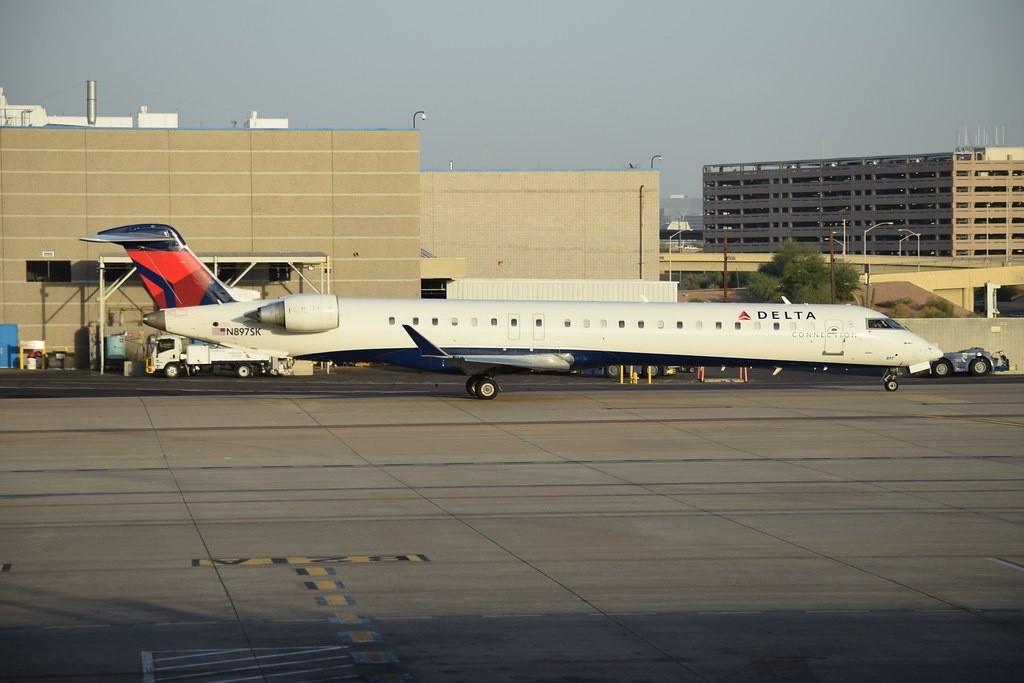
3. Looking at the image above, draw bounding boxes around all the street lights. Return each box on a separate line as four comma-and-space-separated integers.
413, 111, 426, 129
898, 229, 920, 258
670, 230, 682, 281
899, 234, 921, 256
986, 204, 990, 255
843, 220, 847, 254
864, 222, 894, 264
651, 155, 662, 169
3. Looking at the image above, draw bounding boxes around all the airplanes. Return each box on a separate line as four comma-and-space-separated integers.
79, 223, 944, 400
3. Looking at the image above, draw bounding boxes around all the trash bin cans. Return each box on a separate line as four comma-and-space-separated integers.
34, 350, 42, 368
27, 351, 36, 370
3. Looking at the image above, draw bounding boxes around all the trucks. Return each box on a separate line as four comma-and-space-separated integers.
154, 335, 269, 378
